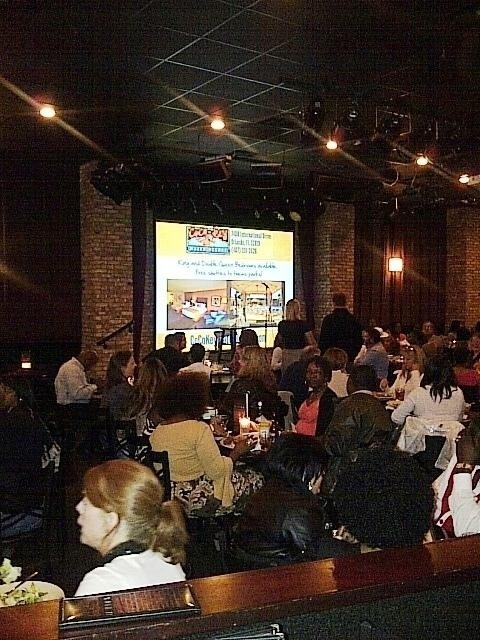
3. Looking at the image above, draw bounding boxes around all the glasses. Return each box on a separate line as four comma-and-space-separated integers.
403, 347, 415, 351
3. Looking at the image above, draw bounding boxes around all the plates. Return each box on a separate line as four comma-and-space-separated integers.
220, 438, 239, 450
0, 581, 64, 606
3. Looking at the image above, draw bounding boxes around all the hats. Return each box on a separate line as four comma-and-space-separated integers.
374, 326, 389, 338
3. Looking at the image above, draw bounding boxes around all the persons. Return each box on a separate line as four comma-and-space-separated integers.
380, 345, 427, 403
317, 293, 364, 362
274, 298, 318, 372
174, 332, 186, 352
323, 449, 436, 549
430, 403, 480, 539
295, 356, 338, 437
324, 365, 398, 456
463, 334, 480, 369
125, 358, 165, 434
323, 348, 352, 398
177, 343, 211, 395
100, 351, 137, 421
0, 372, 46, 539
73, 458, 188, 598
229, 330, 259, 374
52, 350, 98, 430
142, 334, 191, 374
227, 433, 326, 572
421, 320, 446, 358
220, 346, 283, 434
389, 356, 463, 455
148, 373, 265, 518
354, 329, 389, 381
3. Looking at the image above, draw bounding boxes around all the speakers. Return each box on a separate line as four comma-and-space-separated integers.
88, 170, 128, 206
248, 162, 284, 189
305, 172, 359, 205
196, 157, 233, 183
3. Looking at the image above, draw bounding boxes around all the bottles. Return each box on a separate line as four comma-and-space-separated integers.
255, 400, 264, 424
271, 411, 279, 443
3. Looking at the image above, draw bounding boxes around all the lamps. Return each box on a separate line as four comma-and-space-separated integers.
250, 162, 288, 188
371, 106, 407, 146
272, 190, 323, 223
330, 107, 359, 144
307, 171, 349, 200
90, 171, 145, 205
196, 157, 234, 183
366, 198, 398, 225
303, 98, 328, 141
381, 168, 400, 186
425, 118, 460, 151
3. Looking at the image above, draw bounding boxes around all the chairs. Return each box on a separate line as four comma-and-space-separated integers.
106, 418, 147, 457
119, 438, 128, 439
137, 451, 169, 498
1, 461, 55, 577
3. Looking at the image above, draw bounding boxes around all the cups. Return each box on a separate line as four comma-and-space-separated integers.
396, 385, 405, 401
260, 423, 270, 442
239, 417, 250, 433
